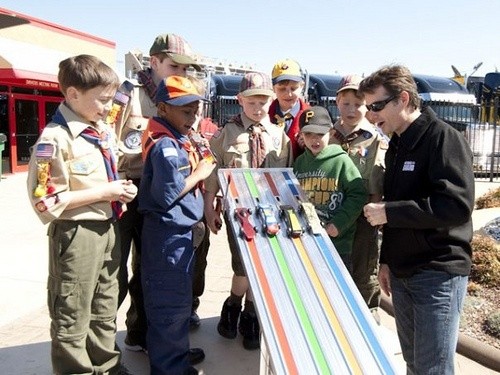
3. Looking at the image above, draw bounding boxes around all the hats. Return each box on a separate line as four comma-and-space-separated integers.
150, 31, 201, 72
240, 72, 273, 98
298, 106, 333, 134
335, 75, 362, 94
271, 59, 303, 85
154, 75, 212, 107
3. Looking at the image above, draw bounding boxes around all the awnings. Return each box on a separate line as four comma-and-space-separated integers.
0, 37, 79, 92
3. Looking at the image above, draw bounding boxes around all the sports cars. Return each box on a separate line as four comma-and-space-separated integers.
233, 206, 259, 242
255, 205, 281, 237
278, 204, 305, 239
298, 201, 324, 236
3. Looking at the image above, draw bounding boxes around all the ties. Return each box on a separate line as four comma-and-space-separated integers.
235, 114, 265, 169
51, 109, 123, 223
333, 128, 363, 154
273, 113, 292, 129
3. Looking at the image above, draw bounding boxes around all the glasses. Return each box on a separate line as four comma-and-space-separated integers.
366, 93, 396, 112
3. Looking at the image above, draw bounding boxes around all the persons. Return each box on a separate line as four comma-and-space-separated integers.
207, 72, 294, 349
136, 75, 217, 375
358, 65, 475, 374
103, 33, 201, 355
290, 106, 366, 278
267, 56, 312, 162
191, 118, 220, 326
27, 54, 139, 375
328, 74, 389, 325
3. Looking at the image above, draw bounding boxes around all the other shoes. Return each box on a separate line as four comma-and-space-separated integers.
191, 311, 200, 326
238, 313, 260, 351
189, 347, 206, 363
125, 335, 148, 354
217, 296, 242, 339
109, 365, 134, 375
185, 365, 199, 375
